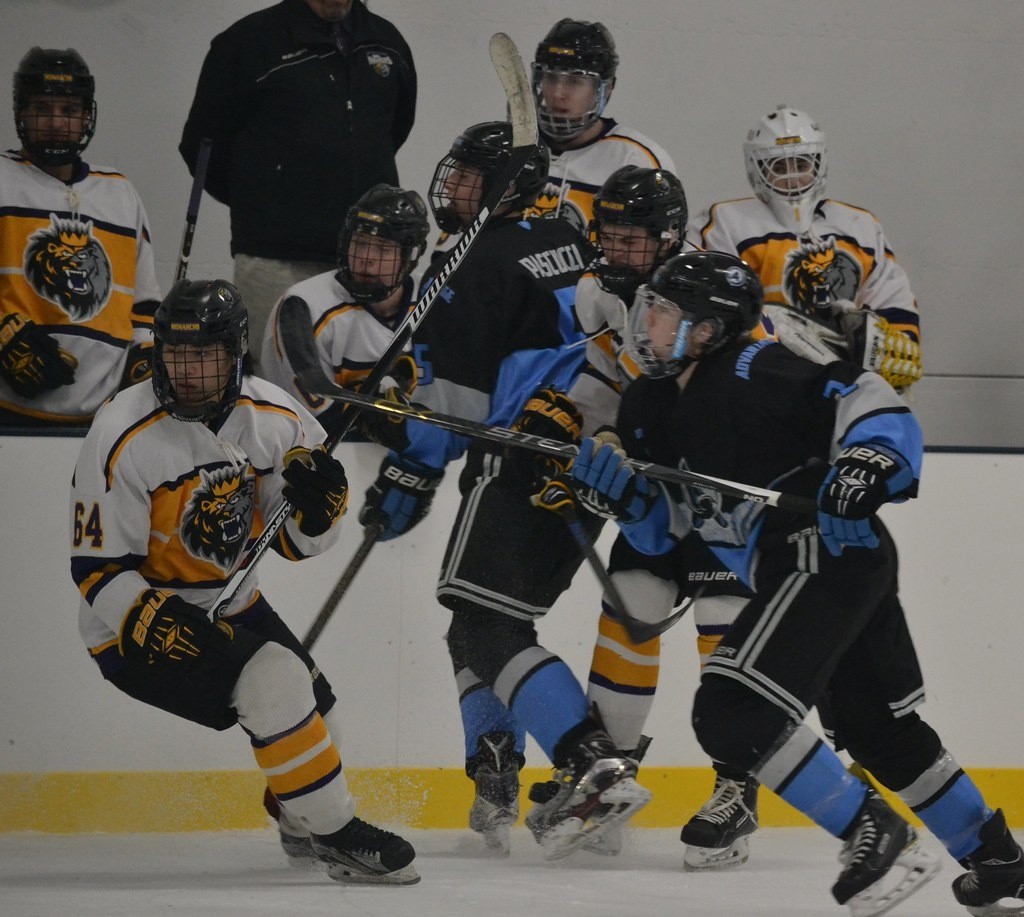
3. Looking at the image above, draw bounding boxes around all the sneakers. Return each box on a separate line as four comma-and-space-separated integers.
950, 807, 1024, 917
469, 731, 525, 858
536, 708, 652, 867
832, 762, 943, 917
680, 760, 761, 873
527, 734, 653, 856
263, 786, 421, 886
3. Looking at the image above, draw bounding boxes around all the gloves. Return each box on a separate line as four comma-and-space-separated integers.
359, 449, 446, 542
500, 385, 586, 499
569, 435, 659, 525
818, 445, 914, 557
1, 311, 77, 400
124, 298, 162, 384
119, 587, 237, 690
281, 445, 349, 537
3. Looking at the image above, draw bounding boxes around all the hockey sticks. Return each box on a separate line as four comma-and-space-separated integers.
277, 292, 819, 514
204, 34, 539, 624
302, 523, 382, 653
175, 138, 213, 281
559, 505, 706, 643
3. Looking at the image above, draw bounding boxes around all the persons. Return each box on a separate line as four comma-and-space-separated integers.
0, 45, 162, 435
362, 110, 654, 856
514, 9, 683, 253
175, 0, 421, 360
256, 179, 431, 439
511, 158, 1024, 917
680, 104, 921, 397
67, 281, 421, 886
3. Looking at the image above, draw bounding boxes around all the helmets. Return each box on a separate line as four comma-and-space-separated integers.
593, 166, 687, 294
153, 279, 249, 422
526, 18, 619, 140
745, 105, 826, 234
11, 46, 98, 164
338, 185, 429, 303
428, 121, 549, 233
622, 250, 764, 379
